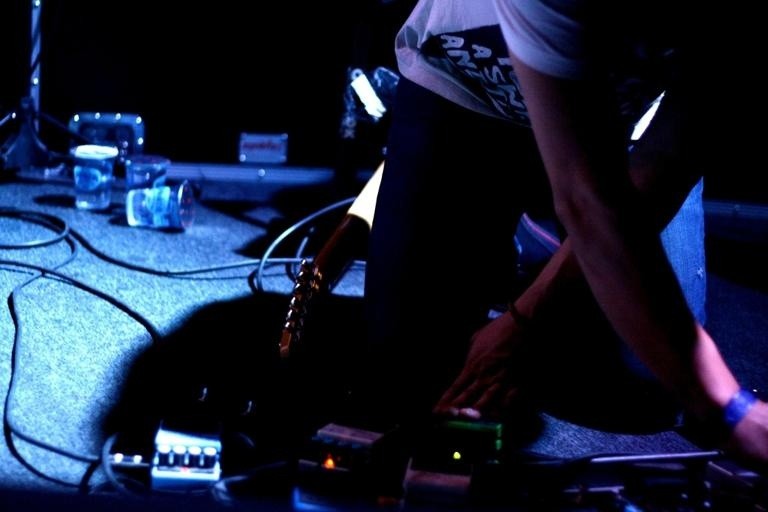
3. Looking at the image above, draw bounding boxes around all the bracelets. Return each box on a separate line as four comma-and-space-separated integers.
501, 305, 543, 340
711, 387, 759, 445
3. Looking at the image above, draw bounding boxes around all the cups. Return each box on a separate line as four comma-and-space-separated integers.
125, 154, 172, 192
125, 180, 199, 232
70, 142, 119, 211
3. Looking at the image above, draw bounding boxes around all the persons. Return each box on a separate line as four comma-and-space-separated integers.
364, 1, 767, 473
